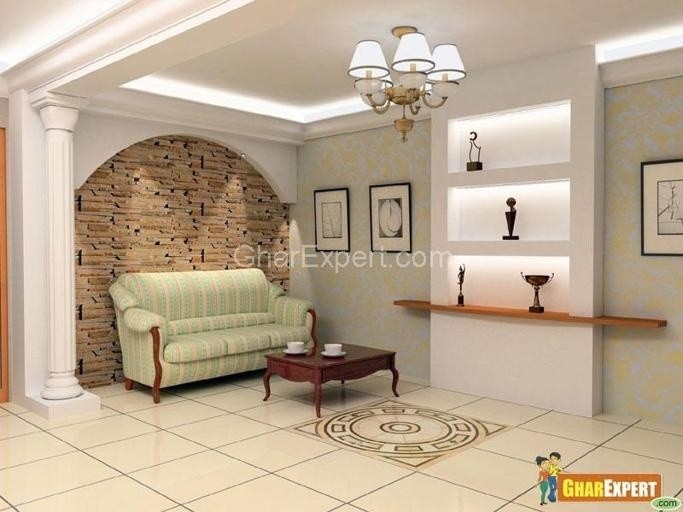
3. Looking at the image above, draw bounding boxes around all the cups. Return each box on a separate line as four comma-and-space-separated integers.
286, 342, 304, 353
323, 344, 343, 355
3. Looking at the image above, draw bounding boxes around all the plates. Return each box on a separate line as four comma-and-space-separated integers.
281, 349, 308, 355
319, 352, 346, 358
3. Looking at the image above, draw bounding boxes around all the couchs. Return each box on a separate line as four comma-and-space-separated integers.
107, 266, 316, 404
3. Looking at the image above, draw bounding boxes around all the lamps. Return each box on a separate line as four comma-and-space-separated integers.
350, 24, 468, 139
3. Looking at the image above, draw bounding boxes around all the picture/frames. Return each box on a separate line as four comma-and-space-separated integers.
312, 187, 352, 253
639, 159, 683, 258
368, 182, 413, 253
